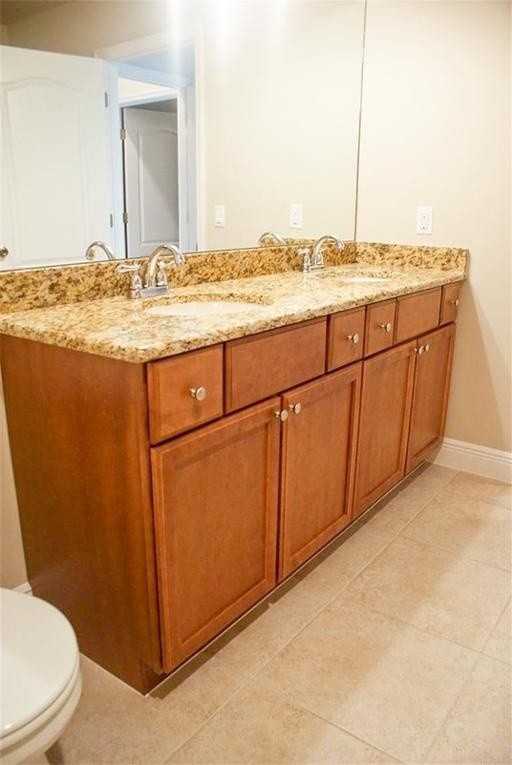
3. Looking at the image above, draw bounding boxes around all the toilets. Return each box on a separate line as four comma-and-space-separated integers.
0, 584, 83, 765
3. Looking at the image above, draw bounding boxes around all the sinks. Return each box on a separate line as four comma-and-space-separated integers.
142, 294, 271, 318
319, 269, 399, 286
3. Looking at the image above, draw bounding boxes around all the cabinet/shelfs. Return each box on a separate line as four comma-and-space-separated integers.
353, 279, 465, 524
1, 306, 366, 701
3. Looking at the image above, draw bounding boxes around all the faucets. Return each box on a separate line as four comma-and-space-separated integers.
313, 235, 346, 264
259, 232, 284, 245
144, 245, 184, 287
85, 241, 115, 262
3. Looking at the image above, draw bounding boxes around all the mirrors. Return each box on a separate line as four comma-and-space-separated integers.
0, 0, 369, 312
